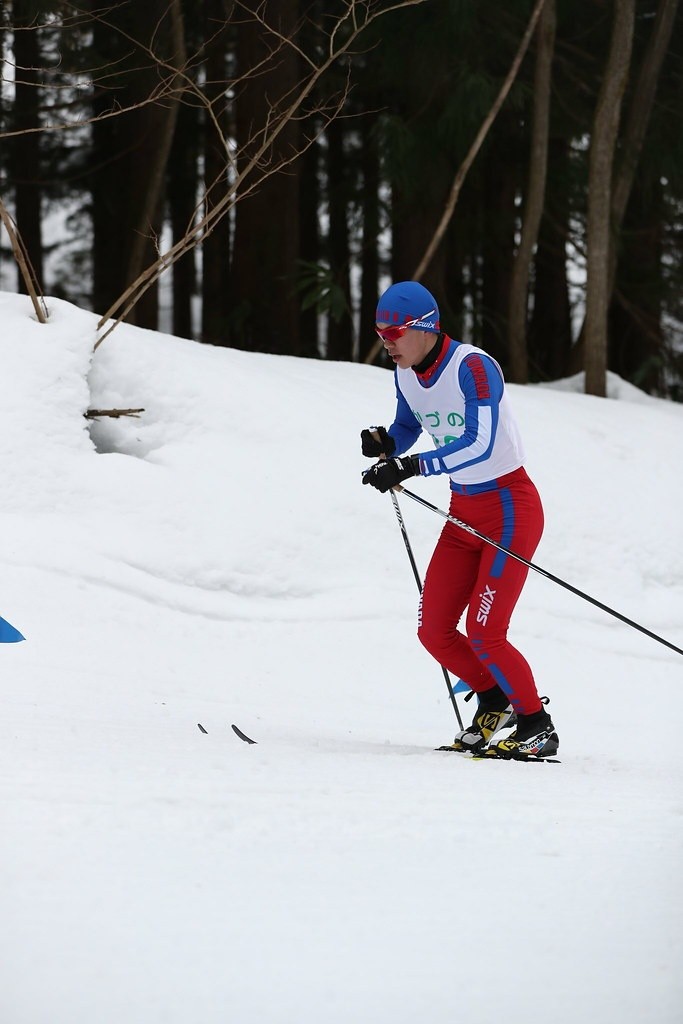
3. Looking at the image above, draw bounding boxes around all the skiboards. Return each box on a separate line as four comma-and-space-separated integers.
199, 720, 562, 766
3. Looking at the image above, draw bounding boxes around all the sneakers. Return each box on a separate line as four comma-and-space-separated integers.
454, 702, 518, 751
488, 714, 559, 757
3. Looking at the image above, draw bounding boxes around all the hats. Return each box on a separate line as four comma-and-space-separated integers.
375, 281, 440, 334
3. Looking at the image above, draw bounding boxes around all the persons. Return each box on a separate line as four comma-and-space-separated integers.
360, 281, 560, 760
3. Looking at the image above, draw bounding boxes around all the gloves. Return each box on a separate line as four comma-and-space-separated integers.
361, 426, 396, 458
362, 456, 417, 493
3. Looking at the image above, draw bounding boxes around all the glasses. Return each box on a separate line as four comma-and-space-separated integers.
374, 323, 410, 343
